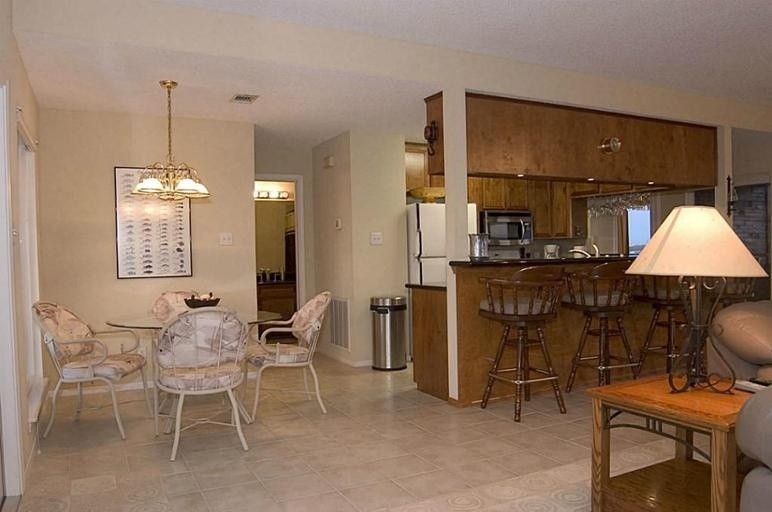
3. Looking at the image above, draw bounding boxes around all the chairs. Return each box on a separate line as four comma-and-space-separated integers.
705, 254, 765, 326
251, 291, 332, 423
153, 291, 200, 316
476, 265, 570, 422
156, 307, 249, 461
562, 273, 641, 393
32, 301, 154, 440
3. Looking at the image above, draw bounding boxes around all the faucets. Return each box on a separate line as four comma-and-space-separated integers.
569, 244, 599, 258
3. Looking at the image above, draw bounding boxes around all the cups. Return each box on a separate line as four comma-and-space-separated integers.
468, 233, 489, 256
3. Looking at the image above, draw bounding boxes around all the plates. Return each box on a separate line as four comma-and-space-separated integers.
469, 256, 490, 263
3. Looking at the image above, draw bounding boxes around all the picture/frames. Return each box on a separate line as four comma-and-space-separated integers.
114, 166, 192, 279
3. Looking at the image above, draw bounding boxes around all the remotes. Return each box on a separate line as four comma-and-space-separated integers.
735, 379, 767, 393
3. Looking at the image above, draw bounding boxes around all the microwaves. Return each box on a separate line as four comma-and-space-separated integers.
481, 210, 534, 245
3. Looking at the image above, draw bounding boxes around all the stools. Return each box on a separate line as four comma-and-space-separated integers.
635, 275, 683, 377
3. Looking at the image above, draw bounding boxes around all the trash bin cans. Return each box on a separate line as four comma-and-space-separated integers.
370, 295, 407, 371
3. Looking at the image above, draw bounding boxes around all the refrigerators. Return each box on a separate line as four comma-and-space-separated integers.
408, 201, 478, 286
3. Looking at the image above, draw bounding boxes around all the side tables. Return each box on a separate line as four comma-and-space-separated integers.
584, 371, 759, 512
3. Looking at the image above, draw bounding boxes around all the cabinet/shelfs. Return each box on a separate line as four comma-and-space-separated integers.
452, 250, 692, 406
571, 180, 599, 197
257, 281, 297, 339
601, 183, 671, 196
469, 174, 528, 210
405, 284, 448, 401
528, 177, 572, 240
402, 140, 445, 195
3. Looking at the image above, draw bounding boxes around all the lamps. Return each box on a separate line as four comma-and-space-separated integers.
625, 206, 770, 393
130, 79, 211, 200
587, 187, 650, 219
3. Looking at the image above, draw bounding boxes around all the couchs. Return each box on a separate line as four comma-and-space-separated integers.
736, 386, 772, 512
706, 298, 772, 385
